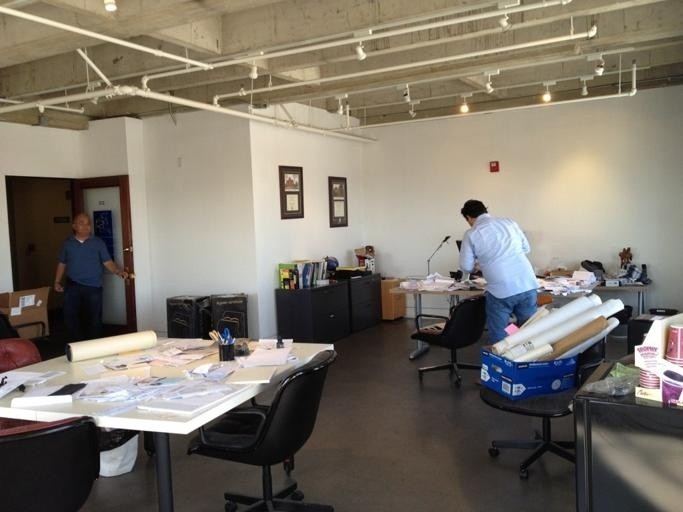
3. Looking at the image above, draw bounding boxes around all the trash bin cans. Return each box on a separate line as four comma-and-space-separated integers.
605, 326, 628, 363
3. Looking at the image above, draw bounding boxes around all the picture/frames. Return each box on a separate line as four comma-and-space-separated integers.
326, 176, 348, 227
278, 165, 304, 221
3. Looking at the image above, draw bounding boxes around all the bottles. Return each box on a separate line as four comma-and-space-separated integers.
642, 264, 648, 279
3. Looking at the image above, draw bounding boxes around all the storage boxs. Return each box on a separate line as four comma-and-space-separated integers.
478, 345, 577, 400
0, 284, 52, 341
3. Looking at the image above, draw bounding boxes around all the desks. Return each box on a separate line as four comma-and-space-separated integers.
0, 336, 337, 512
536, 284, 650, 342
389, 283, 489, 360
571, 349, 682, 511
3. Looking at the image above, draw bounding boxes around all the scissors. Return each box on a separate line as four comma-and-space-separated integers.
209, 328, 231, 345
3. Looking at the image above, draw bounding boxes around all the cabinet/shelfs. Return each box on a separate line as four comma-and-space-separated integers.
274, 281, 349, 344
379, 275, 406, 321
332, 270, 382, 333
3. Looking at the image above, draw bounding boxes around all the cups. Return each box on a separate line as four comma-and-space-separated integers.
661, 369, 683, 408
218, 343, 235, 362
639, 366, 659, 389
665, 325, 683, 367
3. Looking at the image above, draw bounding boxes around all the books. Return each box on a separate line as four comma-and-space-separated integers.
227, 366, 277, 384
279, 261, 328, 290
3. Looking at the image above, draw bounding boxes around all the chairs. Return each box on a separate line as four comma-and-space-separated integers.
0, 335, 42, 373
478, 355, 605, 481
185, 350, 338, 511
409, 294, 488, 388
0, 415, 101, 512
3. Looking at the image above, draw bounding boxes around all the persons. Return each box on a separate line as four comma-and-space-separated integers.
459, 200, 541, 345
54, 214, 128, 354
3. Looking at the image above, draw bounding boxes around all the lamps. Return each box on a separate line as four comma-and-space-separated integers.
425, 235, 450, 276
246, 8, 516, 85
330, 75, 597, 123
